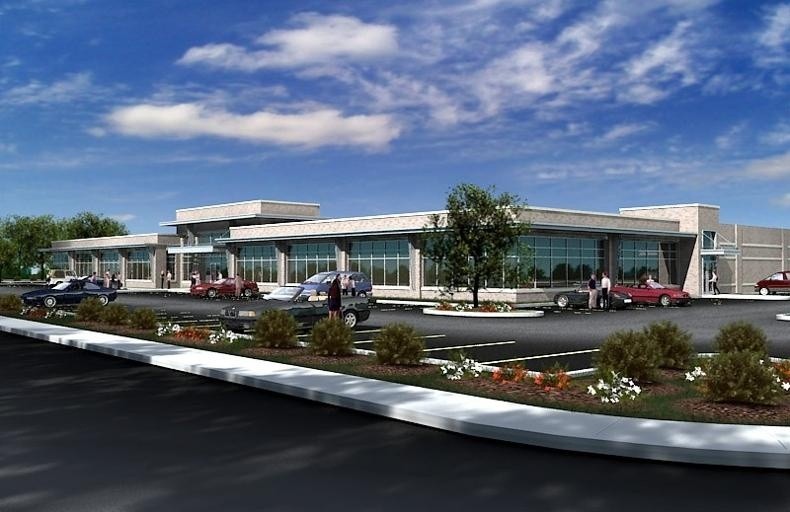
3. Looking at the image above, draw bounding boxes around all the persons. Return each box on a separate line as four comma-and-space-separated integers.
160, 270, 164, 289
646, 273, 654, 283
638, 277, 648, 289
190, 270, 195, 287
217, 271, 222, 280
88, 268, 122, 290
708, 270, 721, 295
46, 270, 52, 285
601, 272, 611, 312
327, 272, 356, 322
192, 270, 201, 285
588, 273, 599, 312
166, 269, 171, 289
234, 273, 243, 299
